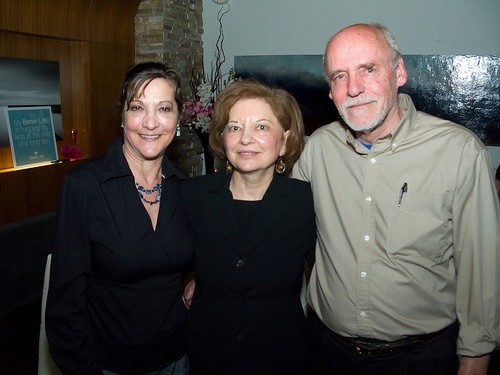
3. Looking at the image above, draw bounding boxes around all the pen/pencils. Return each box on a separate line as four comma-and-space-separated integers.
398, 182, 408, 208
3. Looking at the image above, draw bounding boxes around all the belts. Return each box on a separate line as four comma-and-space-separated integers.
334, 321, 460, 361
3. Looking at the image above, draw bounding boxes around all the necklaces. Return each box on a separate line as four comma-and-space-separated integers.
134, 181, 163, 206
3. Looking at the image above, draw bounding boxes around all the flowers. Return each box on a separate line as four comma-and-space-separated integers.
178, 2, 245, 135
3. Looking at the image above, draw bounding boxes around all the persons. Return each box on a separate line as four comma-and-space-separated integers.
183, 23, 500, 375
44, 62, 193, 375
175, 82, 318, 374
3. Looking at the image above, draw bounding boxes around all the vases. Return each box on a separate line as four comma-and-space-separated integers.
190, 124, 217, 177
